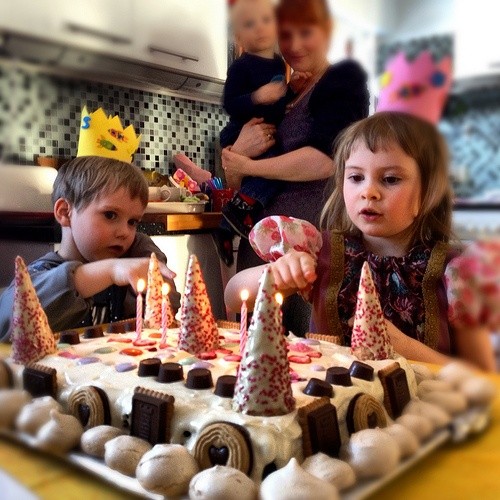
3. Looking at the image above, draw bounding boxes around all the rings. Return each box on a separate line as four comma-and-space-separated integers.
225, 166, 227, 171
268, 134, 273, 141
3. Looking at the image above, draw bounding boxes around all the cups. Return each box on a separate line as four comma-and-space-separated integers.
148, 187, 192, 202
214, 189, 232, 212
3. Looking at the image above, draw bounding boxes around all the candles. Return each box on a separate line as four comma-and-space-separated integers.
240, 289, 250, 348
274, 292, 283, 332
135, 278, 145, 337
161, 282, 171, 343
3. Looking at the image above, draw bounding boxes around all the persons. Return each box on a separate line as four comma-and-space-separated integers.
223, 111, 499, 379
219, 0, 370, 339
0, 156, 181, 344
210, 1, 312, 269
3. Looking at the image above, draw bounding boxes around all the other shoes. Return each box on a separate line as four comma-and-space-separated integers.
220, 193, 254, 239
209, 226, 234, 268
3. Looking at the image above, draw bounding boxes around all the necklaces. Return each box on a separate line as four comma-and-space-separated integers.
287, 68, 324, 109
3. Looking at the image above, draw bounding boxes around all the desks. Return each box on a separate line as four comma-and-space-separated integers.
0, 317, 500, 500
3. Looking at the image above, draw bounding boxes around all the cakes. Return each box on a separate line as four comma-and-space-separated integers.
0, 251, 492, 500
